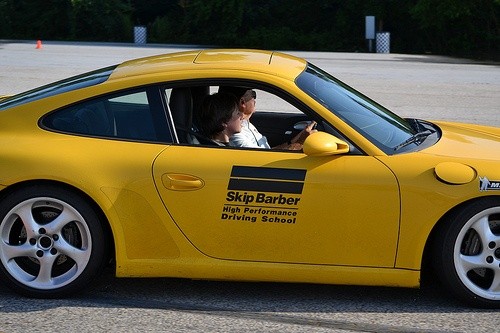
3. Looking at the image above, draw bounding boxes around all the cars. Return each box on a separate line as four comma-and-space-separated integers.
0, 48, 500, 310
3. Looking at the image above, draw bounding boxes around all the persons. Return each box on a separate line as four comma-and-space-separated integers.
218, 87, 317, 149
199, 92, 244, 147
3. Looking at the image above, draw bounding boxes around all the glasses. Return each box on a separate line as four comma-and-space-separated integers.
252, 90, 257, 99
